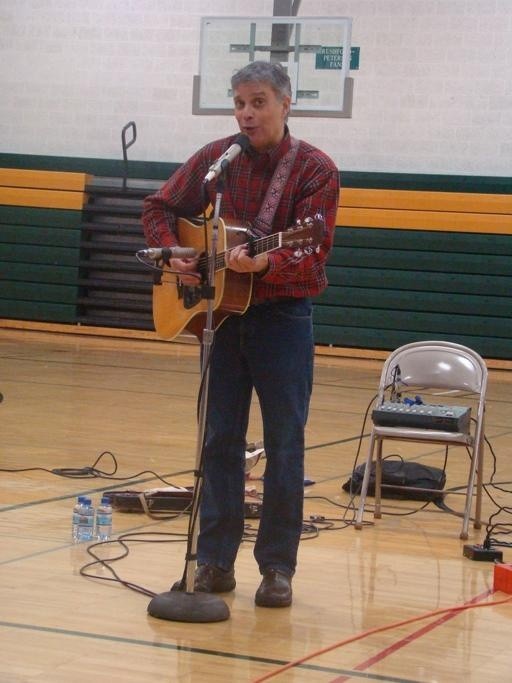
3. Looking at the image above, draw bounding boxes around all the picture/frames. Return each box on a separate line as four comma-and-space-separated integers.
192, 13, 355, 120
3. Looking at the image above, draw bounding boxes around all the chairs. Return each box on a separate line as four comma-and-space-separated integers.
355, 340, 489, 541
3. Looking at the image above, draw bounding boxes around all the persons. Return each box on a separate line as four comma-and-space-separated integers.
139, 59, 341, 608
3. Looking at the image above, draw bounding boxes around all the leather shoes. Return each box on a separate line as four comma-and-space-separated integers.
255, 569, 294, 608
172, 562, 236, 595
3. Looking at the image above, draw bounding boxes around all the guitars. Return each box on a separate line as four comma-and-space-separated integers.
152, 213, 326, 342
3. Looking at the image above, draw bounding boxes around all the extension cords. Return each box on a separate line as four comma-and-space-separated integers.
462, 544, 503, 563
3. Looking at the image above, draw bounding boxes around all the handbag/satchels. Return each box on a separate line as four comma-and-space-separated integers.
343, 459, 448, 501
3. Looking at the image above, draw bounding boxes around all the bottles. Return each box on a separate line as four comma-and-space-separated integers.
73, 498, 86, 538
77, 499, 94, 540
96, 497, 113, 541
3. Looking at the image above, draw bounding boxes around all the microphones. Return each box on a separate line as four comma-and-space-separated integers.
148, 248, 196, 260
201, 133, 252, 186
